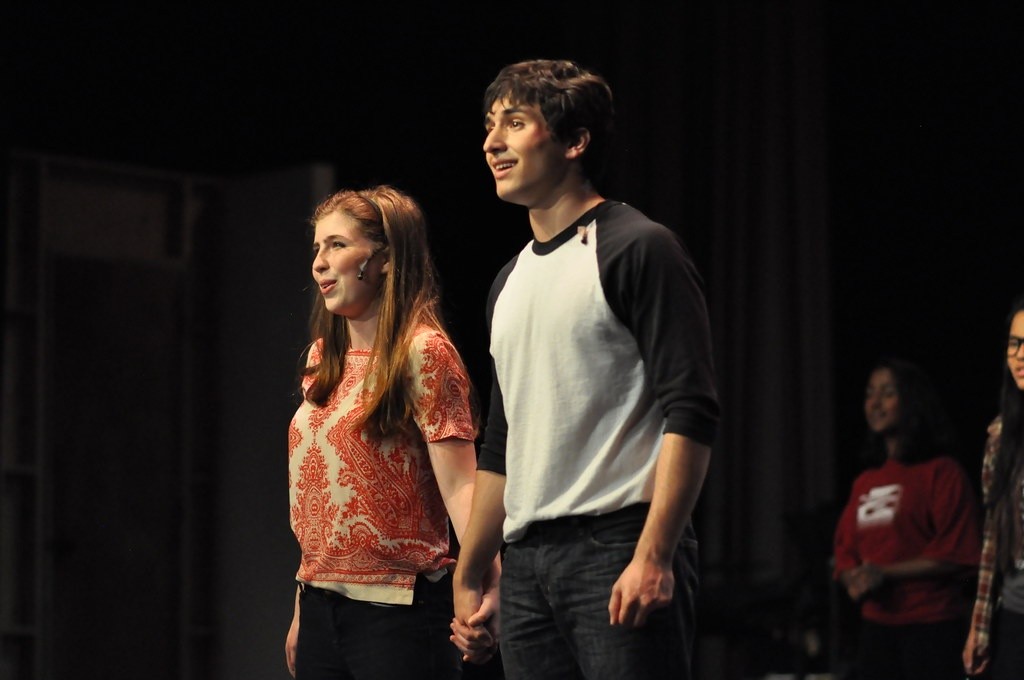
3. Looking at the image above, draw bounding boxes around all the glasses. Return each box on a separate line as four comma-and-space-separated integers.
1006, 336, 1024, 356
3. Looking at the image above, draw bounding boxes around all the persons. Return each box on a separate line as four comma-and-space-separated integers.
830, 311, 1024, 680
282, 60, 718, 680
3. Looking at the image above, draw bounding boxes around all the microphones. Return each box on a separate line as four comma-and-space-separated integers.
358, 247, 384, 280
582, 235, 587, 245
